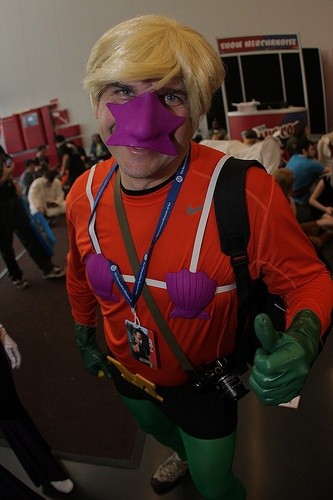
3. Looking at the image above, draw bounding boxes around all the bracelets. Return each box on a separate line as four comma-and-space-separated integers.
0, 324, 6, 337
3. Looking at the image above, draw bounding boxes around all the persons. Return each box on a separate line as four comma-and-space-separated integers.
0, 120, 331, 292
0, 323, 85, 500
65, 14, 333, 500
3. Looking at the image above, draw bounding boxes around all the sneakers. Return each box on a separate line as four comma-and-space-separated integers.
13, 278, 29, 289
151, 452, 190, 490
42, 264, 65, 278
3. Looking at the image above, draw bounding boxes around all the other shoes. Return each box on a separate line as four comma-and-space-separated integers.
51, 478, 74, 492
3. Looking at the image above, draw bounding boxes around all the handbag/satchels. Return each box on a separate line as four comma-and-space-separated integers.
214, 157, 333, 367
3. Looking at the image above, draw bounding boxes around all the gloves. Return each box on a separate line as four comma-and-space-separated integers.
0, 325, 21, 368
73, 321, 113, 379
248, 309, 322, 407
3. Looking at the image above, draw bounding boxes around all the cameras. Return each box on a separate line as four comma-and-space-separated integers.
191, 354, 245, 400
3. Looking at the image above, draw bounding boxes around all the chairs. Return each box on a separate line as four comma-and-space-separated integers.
289, 175, 318, 223
